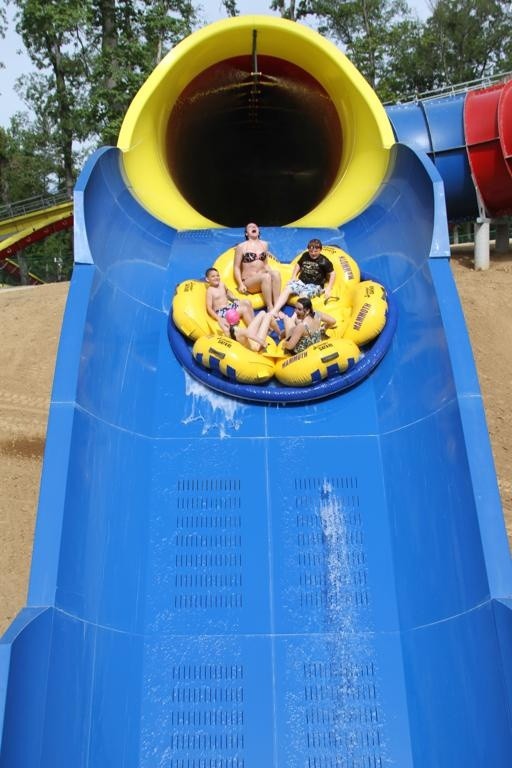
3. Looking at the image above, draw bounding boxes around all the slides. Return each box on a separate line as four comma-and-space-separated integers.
0, 144, 511, 768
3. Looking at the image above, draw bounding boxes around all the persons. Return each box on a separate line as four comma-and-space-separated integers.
225, 308, 284, 354
278, 297, 336, 354
233, 221, 287, 321
268, 238, 335, 319
204, 267, 254, 334
